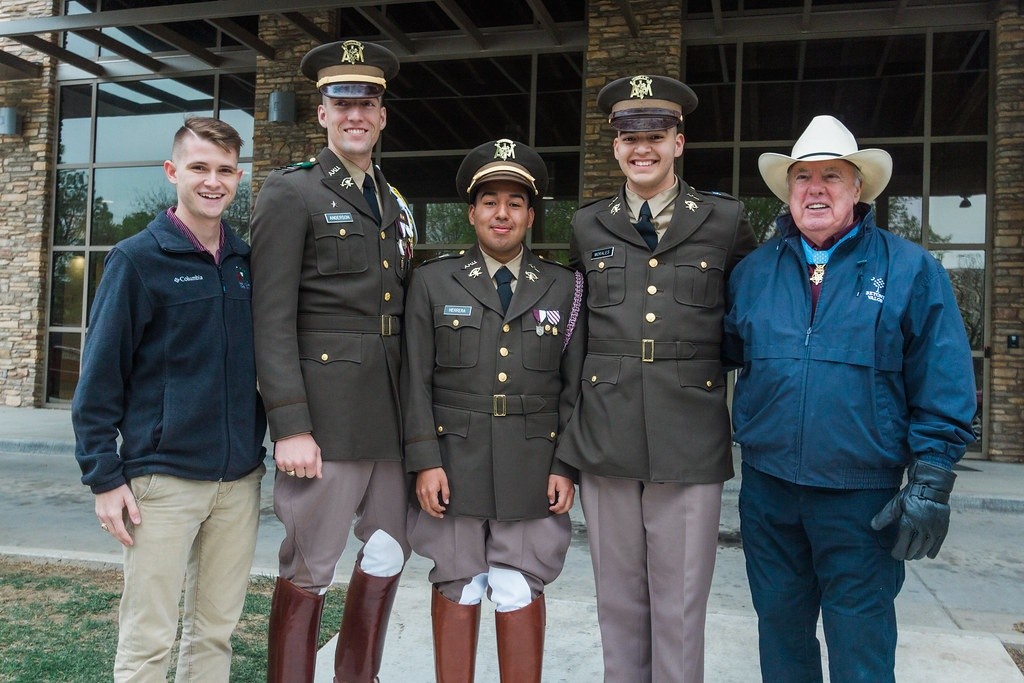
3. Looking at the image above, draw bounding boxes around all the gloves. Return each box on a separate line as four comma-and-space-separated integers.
870, 460, 957, 560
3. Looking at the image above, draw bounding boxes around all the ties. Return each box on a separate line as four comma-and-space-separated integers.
494, 266, 513, 315
362, 173, 382, 227
632, 201, 658, 252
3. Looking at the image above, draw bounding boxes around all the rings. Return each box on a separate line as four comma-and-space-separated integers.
101, 523, 107, 531
287, 470, 294, 475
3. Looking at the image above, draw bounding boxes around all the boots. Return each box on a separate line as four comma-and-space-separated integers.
431, 584, 481, 683
334, 563, 403, 683
496, 595, 547, 683
268, 577, 324, 683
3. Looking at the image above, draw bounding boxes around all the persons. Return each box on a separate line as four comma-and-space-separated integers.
248, 40, 412, 683
568, 75, 760, 683
400, 138, 588, 683
72, 117, 268, 683
725, 115, 978, 683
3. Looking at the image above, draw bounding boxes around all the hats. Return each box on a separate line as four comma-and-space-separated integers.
596, 75, 698, 132
300, 40, 400, 98
759, 115, 893, 205
456, 139, 549, 204
